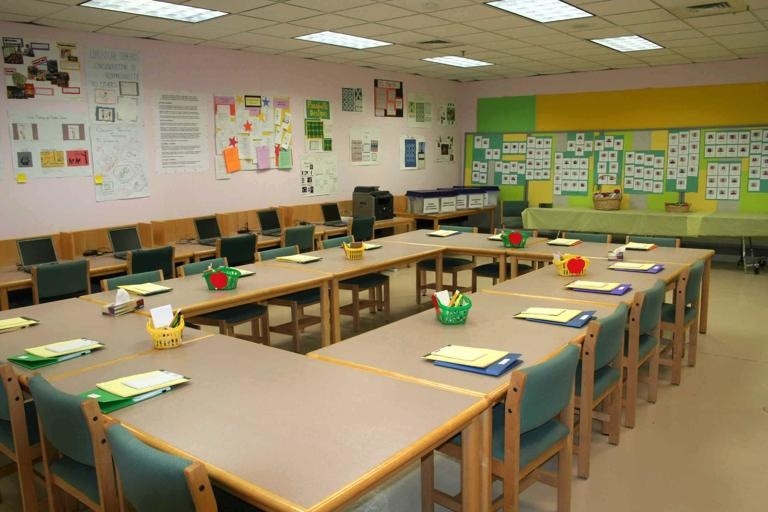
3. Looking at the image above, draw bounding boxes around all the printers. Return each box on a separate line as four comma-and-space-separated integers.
352, 186, 394, 220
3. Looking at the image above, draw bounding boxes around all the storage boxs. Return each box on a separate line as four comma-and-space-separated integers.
452, 185, 500, 208
406, 190, 458, 213
436, 187, 486, 208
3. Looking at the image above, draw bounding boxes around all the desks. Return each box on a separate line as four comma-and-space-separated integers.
0, 296, 216, 387
47, 335, 488, 510
398, 207, 494, 234
80, 243, 450, 351
521, 208, 768, 276
307, 247, 724, 437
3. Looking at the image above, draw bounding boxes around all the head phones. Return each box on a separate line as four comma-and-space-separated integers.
299, 221, 307, 225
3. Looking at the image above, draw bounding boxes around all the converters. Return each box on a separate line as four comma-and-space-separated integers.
83, 248, 97, 257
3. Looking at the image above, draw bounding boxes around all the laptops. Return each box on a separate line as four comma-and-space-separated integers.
107, 225, 144, 260
255, 207, 283, 236
16, 235, 62, 274
192, 215, 222, 246
320, 202, 347, 227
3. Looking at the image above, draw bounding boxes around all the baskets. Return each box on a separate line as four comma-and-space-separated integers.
204, 266, 241, 290
432, 292, 472, 325
593, 198, 621, 210
552, 254, 590, 277
665, 203, 689, 212
500, 230, 528, 248
147, 309, 184, 350
343, 242, 364, 260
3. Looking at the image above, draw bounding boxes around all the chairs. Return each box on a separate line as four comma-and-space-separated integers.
572, 300, 635, 481
13, 215, 376, 311
623, 278, 669, 429
28, 371, 120, 511
411, 223, 682, 312
416, 336, 585, 511
103, 418, 219, 511
659, 260, 706, 387
1, 359, 43, 510
